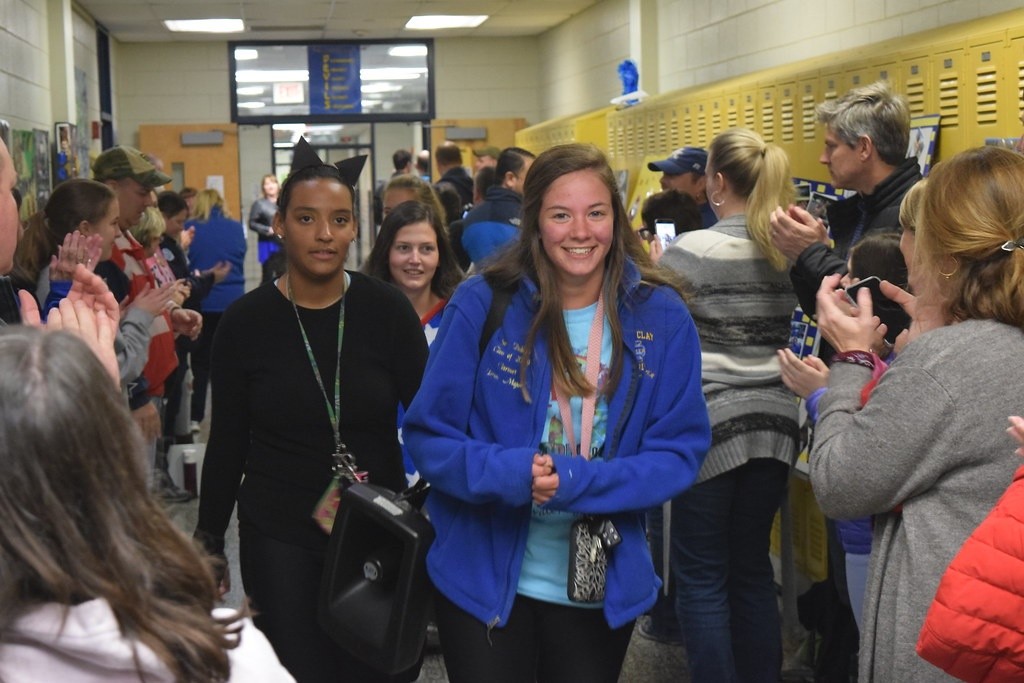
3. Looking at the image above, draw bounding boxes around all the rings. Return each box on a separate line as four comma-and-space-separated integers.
88, 258, 91, 262
773, 232, 776, 237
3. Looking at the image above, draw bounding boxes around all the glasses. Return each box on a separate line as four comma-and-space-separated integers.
639, 227, 652, 240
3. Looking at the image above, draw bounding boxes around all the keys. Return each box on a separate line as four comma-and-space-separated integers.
590, 514, 622, 564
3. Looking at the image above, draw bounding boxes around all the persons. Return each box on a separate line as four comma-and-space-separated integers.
0, 139, 534, 683
459, 144, 538, 273
769, 80, 923, 369
194, 165, 430, 683
635, 126, 800, 683
915, 415, 1024, 683
185, 187, 247, 434
249, 174, 287, 288
777, 130, 1024, 683
0, 323, 296, 683
404, 143, 712, 682
365, 202, 466, 348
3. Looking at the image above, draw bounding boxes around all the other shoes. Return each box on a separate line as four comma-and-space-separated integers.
191, 421, 200, 431
639, 616, 682, 645
151, 477, 194, 502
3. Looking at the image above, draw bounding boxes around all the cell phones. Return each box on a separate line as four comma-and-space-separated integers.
654, 217, 677, 254
845, 276, 911, 350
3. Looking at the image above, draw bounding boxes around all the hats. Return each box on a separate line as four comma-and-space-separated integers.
472, 146, 501, 159
648, 147, 707, 176
94, 144, 172, 186
